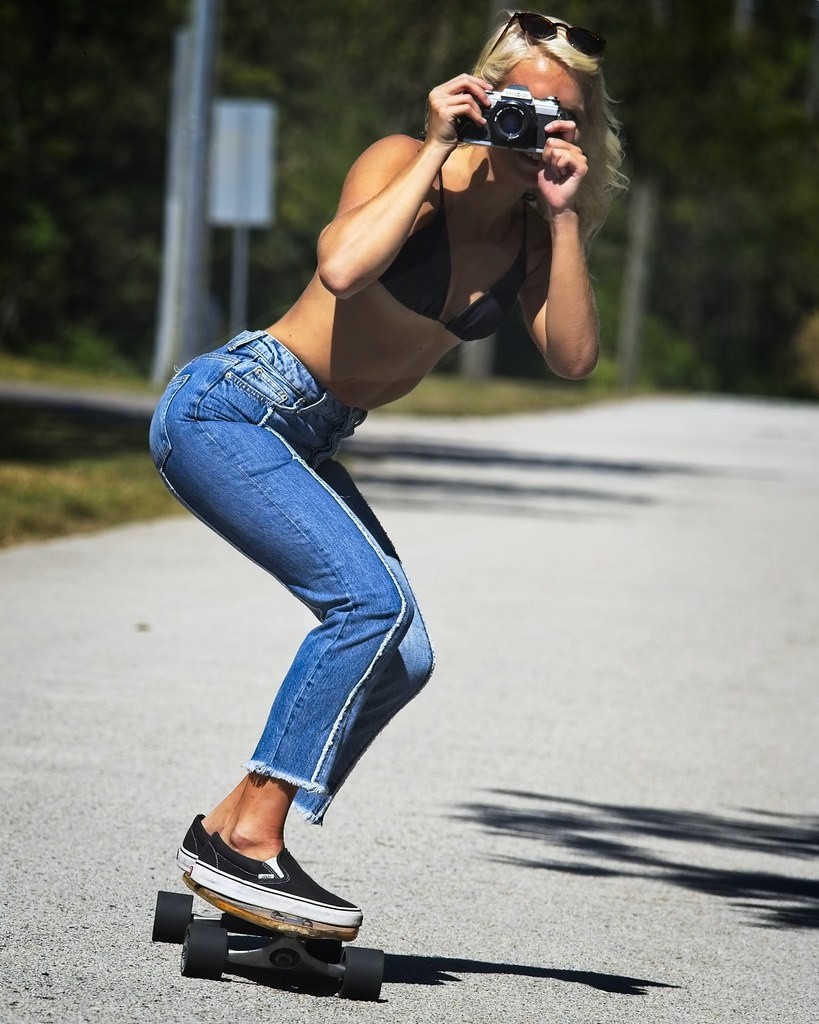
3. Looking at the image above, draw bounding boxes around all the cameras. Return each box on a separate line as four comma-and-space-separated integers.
457, 83, 562, 155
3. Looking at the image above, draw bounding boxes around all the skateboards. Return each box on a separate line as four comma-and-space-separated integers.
151, 870, 386, 1003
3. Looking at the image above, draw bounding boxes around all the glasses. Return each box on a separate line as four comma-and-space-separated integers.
486, 11, 610, 66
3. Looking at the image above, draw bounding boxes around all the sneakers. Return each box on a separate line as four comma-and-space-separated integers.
191, 832, 363, 926
178, 813, 218, 868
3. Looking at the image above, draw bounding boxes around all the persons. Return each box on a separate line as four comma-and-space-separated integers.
149, 11, 629, 930
